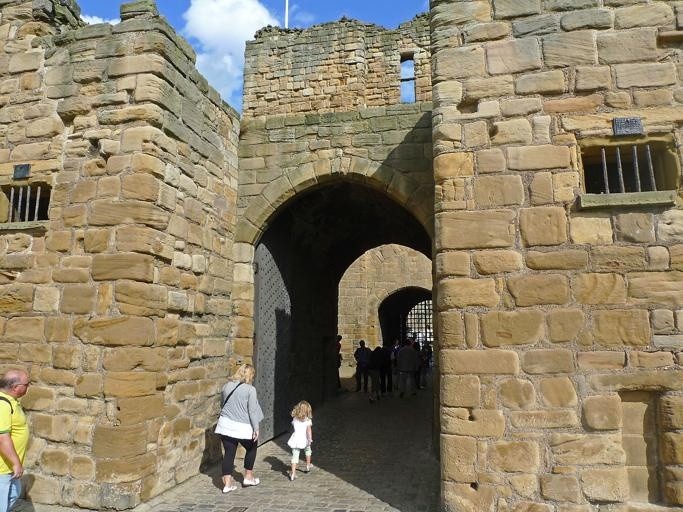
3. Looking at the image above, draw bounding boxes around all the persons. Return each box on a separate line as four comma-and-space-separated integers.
286, 400, 313, 482
337, 334, 342, 390
213, 363, 265, 495
353, 338, 433, 405
0, 367, 30, 511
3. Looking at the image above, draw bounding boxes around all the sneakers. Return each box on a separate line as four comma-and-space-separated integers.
243, 478, 260, 486
223, 486, 237, 493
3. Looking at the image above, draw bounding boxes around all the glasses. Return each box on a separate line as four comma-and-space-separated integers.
18, 383, 30, 387
